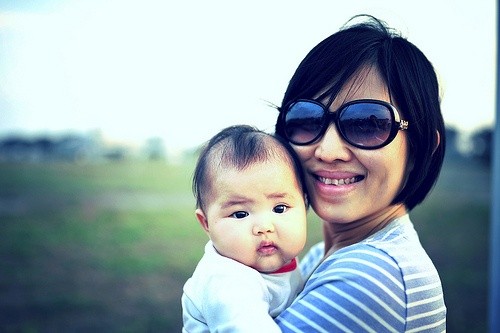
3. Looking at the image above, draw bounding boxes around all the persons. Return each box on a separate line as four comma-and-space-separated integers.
272, 10, 448, 333
180, 123, 308, 333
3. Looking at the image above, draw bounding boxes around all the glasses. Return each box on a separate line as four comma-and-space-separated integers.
280, 98, 424, 150
